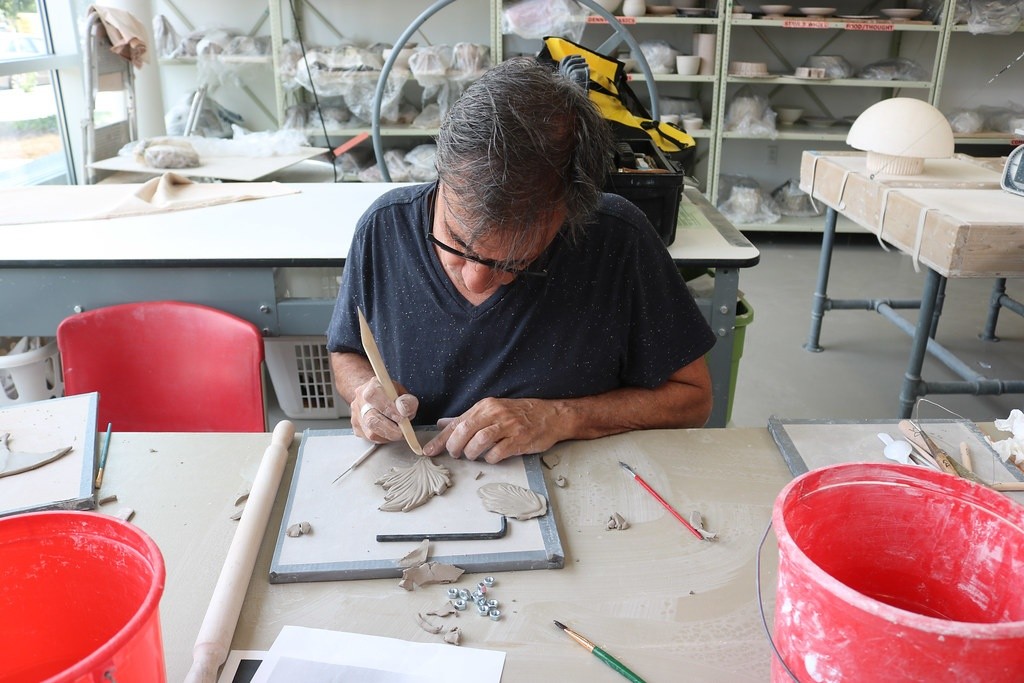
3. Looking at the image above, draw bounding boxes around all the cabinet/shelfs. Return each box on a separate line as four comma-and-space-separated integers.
155, 0, 1024, 234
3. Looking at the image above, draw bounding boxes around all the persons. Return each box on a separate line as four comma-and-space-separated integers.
324, 56, 717, 466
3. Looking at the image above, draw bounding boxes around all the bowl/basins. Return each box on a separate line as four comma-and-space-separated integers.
657, 95, 703, 119
576, 0, 622, 15
382, 49, 417, 71
617, 59, 636, 72
761, 6, 792, 17
774, 107, 804, 124
805, 56, 852, 78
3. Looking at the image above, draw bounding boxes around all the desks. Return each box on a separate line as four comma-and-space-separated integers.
92, 159, 345, 184
0, 184, 763, 428
797, 148, 1024, 421
91, 423, 1024, 683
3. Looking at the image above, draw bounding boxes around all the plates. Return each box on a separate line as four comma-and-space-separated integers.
802, 117, 837, 127
880, 9, 923, 20
844, 118, 856, 124
839, 15, 880, 19
799, 8, 837, 18
648, 6, 676, 14
677, 8, 709, 15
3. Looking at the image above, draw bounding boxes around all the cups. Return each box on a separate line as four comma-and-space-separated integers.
693, 33, 716, 75
676, 56, 701, 75
623, 0, 646, 16
660, 113, 703, 130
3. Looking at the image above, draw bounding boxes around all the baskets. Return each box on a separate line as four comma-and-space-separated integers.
0, 334, 65, 405
264, 337, 350, 419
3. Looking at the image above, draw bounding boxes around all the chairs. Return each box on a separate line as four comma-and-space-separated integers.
57, 302, 269, 431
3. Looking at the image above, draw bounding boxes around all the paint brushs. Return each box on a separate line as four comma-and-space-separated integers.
619, 461, 704, 540
95, 423, 112, 489
553, 619, 646, 683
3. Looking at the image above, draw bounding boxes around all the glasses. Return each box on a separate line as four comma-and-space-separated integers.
427, 179, 568, 281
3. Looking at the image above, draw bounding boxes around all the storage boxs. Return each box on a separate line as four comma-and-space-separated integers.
0, 336, 66, 408
261, 334, 351, 420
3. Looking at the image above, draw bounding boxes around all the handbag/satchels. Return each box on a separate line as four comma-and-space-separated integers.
540, 36, 697, 159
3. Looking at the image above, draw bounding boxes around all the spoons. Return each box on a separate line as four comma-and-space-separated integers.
884, 441, 912, 465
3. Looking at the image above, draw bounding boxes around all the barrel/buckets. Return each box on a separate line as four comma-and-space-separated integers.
0, 510, 166, 683
212, 159, 344, 183
757, 461, 1024, 683
